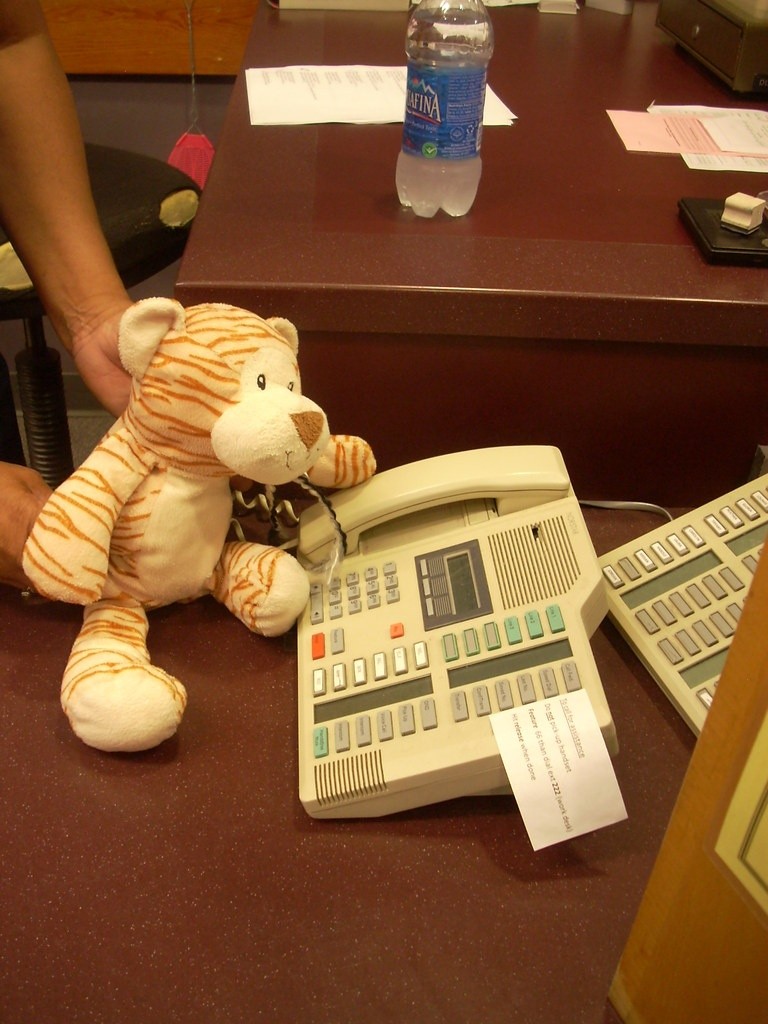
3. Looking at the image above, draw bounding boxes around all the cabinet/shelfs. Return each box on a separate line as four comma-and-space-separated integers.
655, 0, 768, 93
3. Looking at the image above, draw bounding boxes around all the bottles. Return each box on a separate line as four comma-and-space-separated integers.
396, 1, 495, 218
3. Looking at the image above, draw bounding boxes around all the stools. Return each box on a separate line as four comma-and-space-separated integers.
0, 142, 205, 493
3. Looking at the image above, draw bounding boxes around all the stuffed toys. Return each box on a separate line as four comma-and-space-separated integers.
21, 300, 376, 752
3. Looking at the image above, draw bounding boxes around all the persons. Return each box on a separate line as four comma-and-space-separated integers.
0, 0, 130, 593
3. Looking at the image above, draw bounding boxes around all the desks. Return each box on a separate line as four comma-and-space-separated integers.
175, 0, 767, 509
0, 507, 716, 1024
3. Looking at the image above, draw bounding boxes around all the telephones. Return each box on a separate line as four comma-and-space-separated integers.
297, 444, 617, 819
